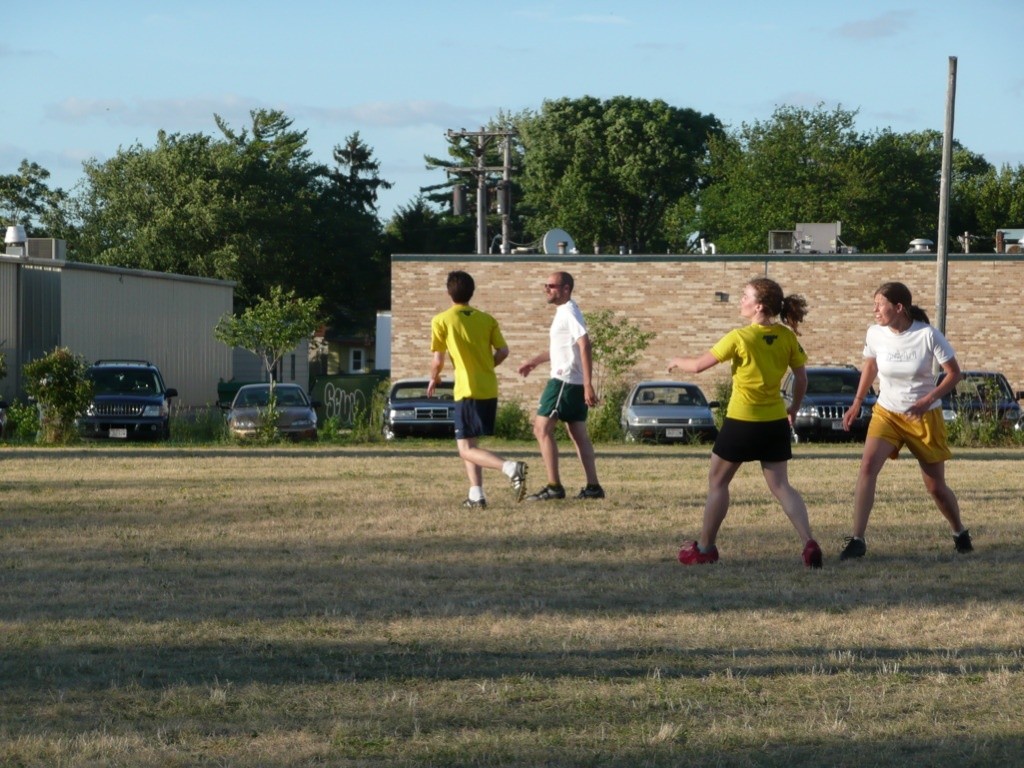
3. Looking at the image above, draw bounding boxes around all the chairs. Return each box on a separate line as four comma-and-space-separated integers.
679, 395, 691, 404
642, 391, 655, 400
283, 394, 297, 402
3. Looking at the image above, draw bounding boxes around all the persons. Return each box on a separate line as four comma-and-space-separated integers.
667, 279, 825, 569
518, 271, 607, 502
838, 284, 974, 558
427, 272, 527, 508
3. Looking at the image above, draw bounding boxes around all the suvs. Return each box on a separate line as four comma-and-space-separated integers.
779, 363, 877, 442
78, 358, 179, 442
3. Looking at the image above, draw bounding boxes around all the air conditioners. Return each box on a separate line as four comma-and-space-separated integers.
25, 238, 66, 261
767, 230, 795, 254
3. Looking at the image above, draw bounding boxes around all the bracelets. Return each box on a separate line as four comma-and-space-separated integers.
433, 375, 439, 379
855, 397, 864, 402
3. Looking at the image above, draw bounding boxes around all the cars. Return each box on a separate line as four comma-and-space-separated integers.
931, 371, 1024, 433
621, 381, 720, 443
218, 383, 321, 442
380, 377, 454, 437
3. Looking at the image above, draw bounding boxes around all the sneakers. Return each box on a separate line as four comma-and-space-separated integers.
839, 536, 866, 559
679, 541, 718, 565
460, 499, 486, 510
804, 540, 822, 568
526, 485, 566, 500
511, 462, 529, 502
574, 485, 605, 500
953, 530, 972, 554
